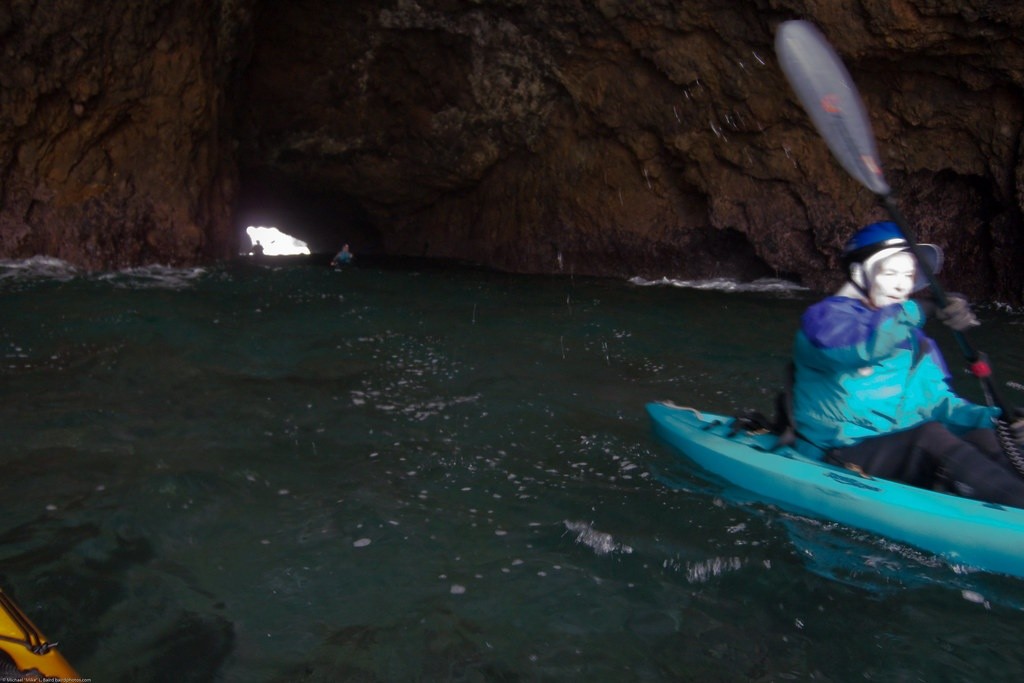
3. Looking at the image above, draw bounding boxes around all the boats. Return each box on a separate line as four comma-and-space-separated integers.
644, 399, 1024, 580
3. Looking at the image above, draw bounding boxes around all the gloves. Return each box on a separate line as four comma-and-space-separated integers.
998, 407, 1024, 447
932, 289, 983, 332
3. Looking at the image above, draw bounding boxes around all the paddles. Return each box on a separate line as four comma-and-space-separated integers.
776, 18, 1024, 475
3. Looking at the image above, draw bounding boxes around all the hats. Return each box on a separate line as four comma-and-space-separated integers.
840, 220, 911, 282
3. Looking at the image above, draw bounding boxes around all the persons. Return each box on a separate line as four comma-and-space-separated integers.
786, 221, 1024, 510
330, 240, 353, 267
253, 240, 264, 254
